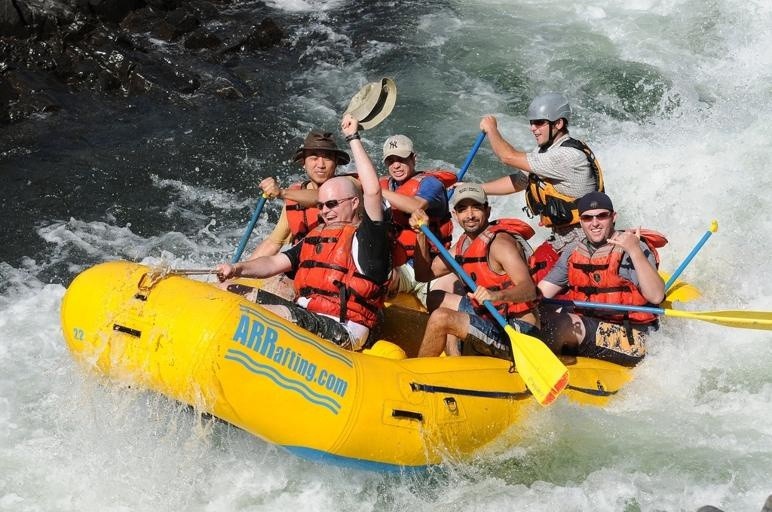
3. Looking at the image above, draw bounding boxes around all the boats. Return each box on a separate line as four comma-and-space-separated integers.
60, 260, 703, 480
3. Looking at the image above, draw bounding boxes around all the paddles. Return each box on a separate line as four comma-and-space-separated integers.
416, 220, 569, 406
657, 271, 705, 303
541, 299, 772, 331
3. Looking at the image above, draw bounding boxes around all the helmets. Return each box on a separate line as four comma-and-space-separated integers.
527, 93, 571, 123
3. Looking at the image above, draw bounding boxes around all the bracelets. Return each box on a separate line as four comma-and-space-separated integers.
345, 131, 360, 142
232, 265, 236, 277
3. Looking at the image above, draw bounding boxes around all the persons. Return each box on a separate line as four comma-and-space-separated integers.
408, 182, 541, 358
378, 134, 454, 294
446, 93, 604, 254
217, 113, 391, 352
248, 128, 350, 301
536, 191, 665, 366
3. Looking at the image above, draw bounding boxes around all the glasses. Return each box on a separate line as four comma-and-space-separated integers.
316, 197, 354, 210
581, 211, 611, 221
530, 120, 545, 125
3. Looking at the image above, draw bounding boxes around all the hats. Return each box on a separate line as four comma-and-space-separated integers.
291, 129, 349, 165
577, 191, 613, 211
453, 183, 488, 208
343, 77, 397, 130
382, 134, 413, 163
